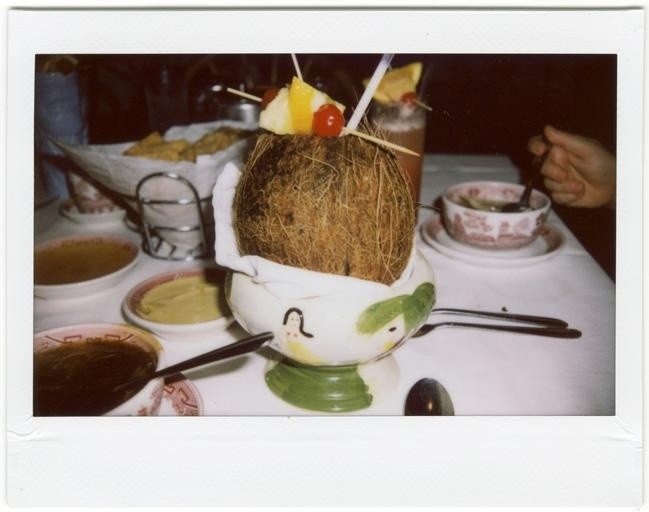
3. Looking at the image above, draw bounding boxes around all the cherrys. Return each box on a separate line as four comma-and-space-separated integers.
313, 103, 346, 138
402, 93, 418, 108
260, 88, 279, 109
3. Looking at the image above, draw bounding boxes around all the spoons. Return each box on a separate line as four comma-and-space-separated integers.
411, 322, 582, 340
404, 377, 455, 415
501, 143, 550, 213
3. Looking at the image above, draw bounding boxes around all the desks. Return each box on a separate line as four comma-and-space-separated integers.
34, 152, 615, 415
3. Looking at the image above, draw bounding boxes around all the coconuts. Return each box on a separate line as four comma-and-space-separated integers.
232, 76, 416, 285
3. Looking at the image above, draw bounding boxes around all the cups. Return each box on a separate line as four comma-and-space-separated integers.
367, 103, 427, 230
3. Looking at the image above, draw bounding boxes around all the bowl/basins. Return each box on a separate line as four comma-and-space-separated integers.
62, 164, 119, 216
33, 322, 168, 416
440, 181, 551, 251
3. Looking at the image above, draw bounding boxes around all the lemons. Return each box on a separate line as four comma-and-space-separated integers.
361, 61, 422, 103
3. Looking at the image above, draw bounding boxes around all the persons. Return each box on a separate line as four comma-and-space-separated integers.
525, 125, 616, 210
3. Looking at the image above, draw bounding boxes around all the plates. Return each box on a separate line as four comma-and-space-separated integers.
59, 198, 128, 226
420, 215, 567, 268
34, 233, 142, 301
155, 364, 204, 417
121, 264, 236, 340
